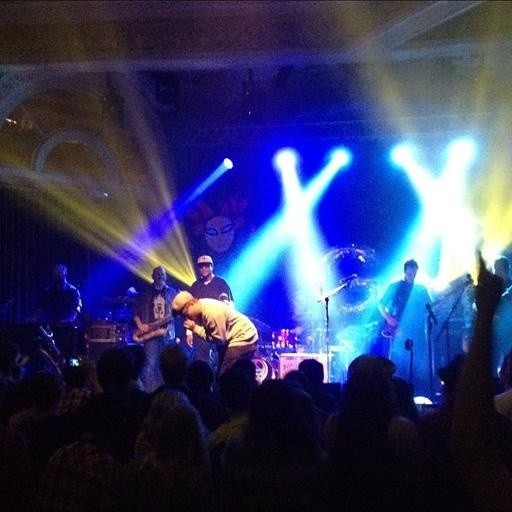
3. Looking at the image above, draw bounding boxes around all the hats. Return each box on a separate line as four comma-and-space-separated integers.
171, 290, 192, 317
197, 255, 213, 264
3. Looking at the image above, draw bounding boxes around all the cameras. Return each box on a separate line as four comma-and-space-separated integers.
68, 356, 81, 368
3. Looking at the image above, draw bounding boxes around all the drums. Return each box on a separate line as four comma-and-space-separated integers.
252, 358, 276, 384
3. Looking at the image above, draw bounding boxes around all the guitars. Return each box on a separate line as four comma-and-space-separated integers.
382, 275, 473, 344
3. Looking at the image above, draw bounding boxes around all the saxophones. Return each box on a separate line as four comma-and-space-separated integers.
133, 314, 173, 343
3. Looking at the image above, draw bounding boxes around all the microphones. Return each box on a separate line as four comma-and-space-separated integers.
340, 274, 357, 284
426, 303, 437, 321
466, 274, 473, 284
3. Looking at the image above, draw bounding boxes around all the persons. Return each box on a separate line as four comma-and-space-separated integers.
1, 255, 512, 512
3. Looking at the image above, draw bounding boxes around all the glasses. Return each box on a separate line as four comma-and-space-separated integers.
198, 262, 210, 268
